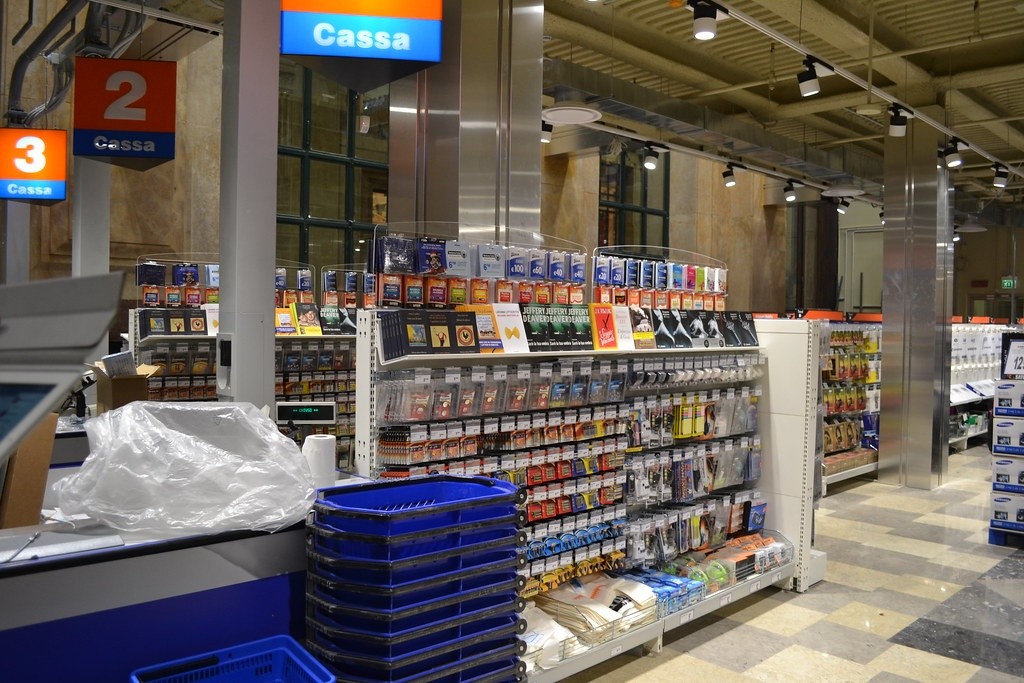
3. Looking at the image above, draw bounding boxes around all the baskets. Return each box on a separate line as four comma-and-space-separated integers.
305, 475, 527, 683
129, 634, 336, 683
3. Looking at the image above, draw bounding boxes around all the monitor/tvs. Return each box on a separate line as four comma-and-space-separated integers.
0, 370, 83, 469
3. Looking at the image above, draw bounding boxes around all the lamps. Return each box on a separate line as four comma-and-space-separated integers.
797, 55, 836, 97
943, 135, 969, 168
990, 162, 1009, 188
878, 207, 885, 225
887, 102, 915, 138
685, 0, 732, 41
722, 163, 748, 187
783, 178, 805, 202
642, 143, 670, 170
837, 196, 855, 215
540, 120, 554, 143
953, 225, 961, 242
